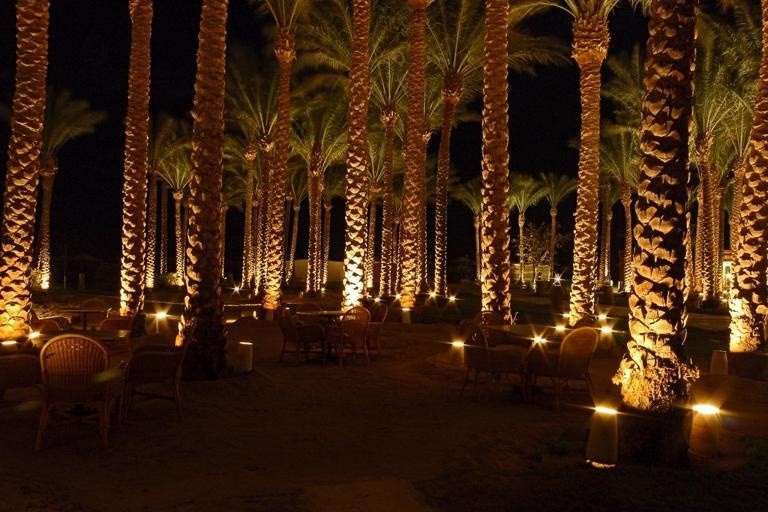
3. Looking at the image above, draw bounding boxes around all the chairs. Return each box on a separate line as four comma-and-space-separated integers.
329, 306, 370, 366
367, 302, 390, 358
296, 300, 321, 341
533, 330, 599, 408
476, 310, 506, 335
30, 309, 71, 335
119, 319, 196, 415
38, 333, 114, 445
102, 299, 141, 334
275, 305, 326, 363
457, 318, 527, 405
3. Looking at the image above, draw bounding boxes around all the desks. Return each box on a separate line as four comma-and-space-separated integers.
40, 331, 122, 354
58, 308, 100, 332
296, 308, 348, 325
493, 323, 570, 396
